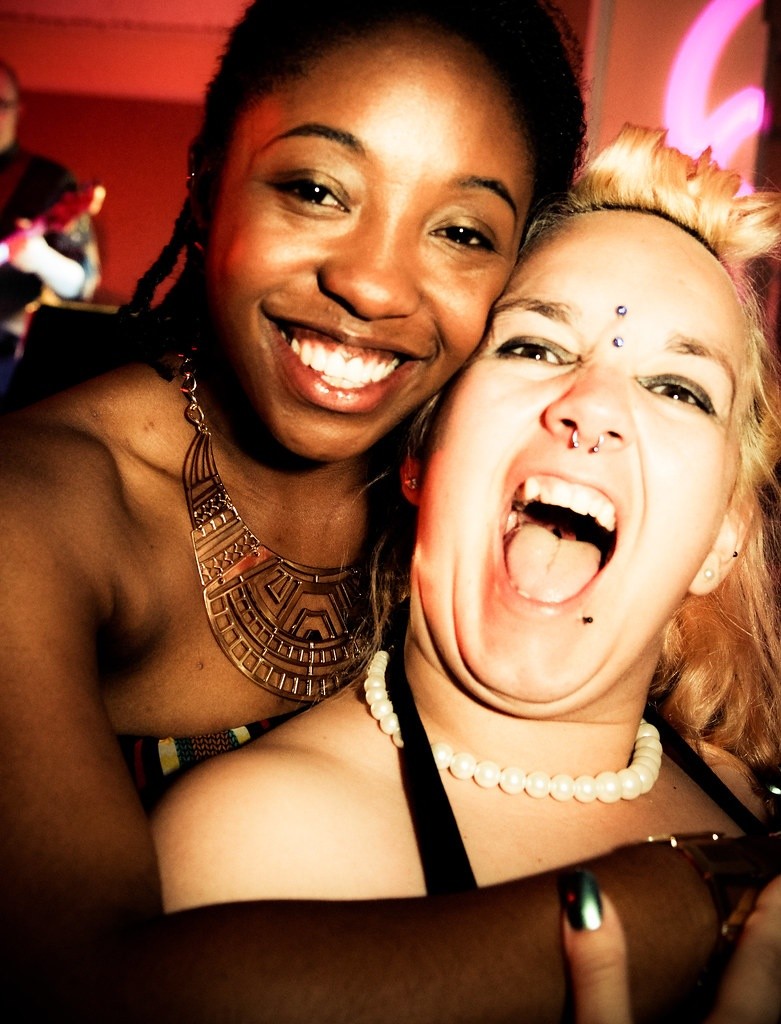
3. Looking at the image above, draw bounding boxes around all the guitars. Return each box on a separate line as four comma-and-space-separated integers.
0, 180, 106, 268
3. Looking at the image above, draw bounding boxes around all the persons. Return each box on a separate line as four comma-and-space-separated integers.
0, 0, 780, 1024
0, 56, 105, 398
150, 122, 781, 1024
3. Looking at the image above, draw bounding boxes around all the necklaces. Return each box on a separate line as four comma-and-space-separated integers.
363, 648, 663, 804
165, 337, 396, 703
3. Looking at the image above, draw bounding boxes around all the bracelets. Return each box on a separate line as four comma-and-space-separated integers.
671, 820, 761, 1013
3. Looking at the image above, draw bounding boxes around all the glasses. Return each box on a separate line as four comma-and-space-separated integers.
1, 98, 18, 110
